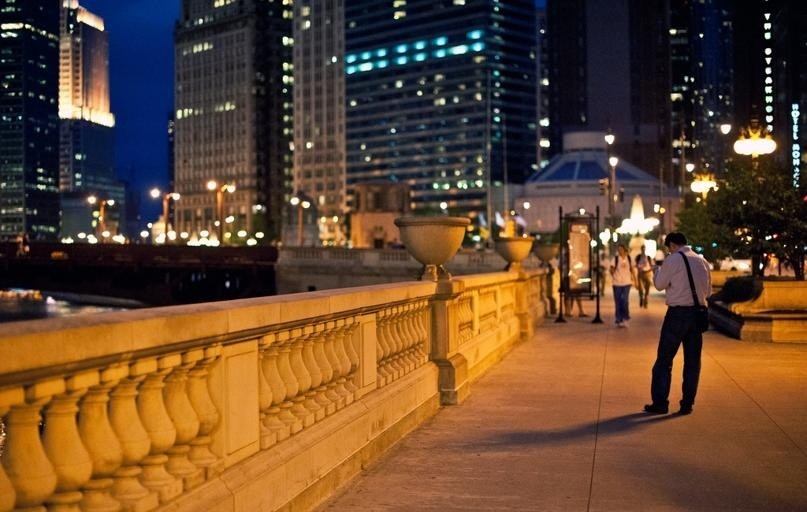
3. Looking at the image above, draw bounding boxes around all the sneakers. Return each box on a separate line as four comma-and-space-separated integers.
615, 318, 631, 328
639, 303, 648, 309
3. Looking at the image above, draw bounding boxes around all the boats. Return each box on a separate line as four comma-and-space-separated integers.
0, 287, 46, 318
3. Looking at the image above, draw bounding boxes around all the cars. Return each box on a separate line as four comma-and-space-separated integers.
719, 251, 752, 273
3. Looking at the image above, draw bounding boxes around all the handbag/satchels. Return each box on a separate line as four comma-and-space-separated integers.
701, 305, 709, 333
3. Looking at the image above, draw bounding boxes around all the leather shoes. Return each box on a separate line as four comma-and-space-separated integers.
645, 402, 668, 414
678, 400, 693, 414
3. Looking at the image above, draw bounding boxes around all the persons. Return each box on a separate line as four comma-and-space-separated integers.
644, 233, 713, 414
609, 244, 638, 328
634, 246, 655, 308
596, 253, 610, 297
563, 297, 590, 318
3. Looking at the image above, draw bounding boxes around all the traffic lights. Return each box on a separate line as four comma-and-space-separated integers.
598, 179, 605, 195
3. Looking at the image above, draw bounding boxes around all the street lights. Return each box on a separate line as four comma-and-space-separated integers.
86, 195, 114, 243
288, 193, 310, 248
151, 189, 181, 245
605, 125, 617, 226
656, 120, 777, 249
206, 180, 235, 246
